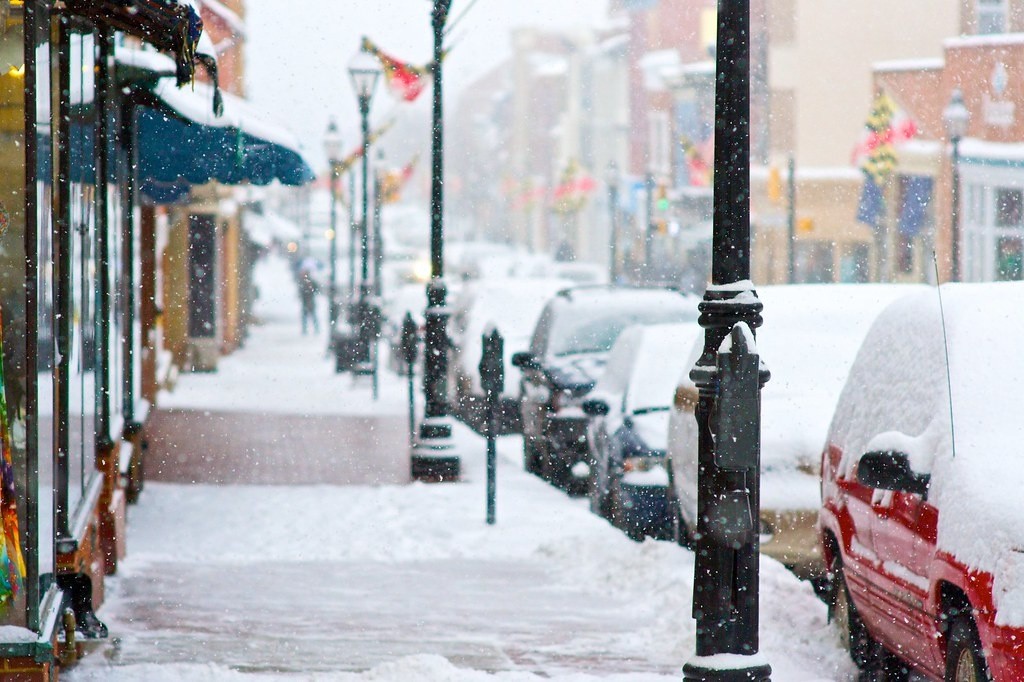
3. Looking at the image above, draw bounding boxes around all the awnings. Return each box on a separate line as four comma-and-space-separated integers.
135, 80, 320, 202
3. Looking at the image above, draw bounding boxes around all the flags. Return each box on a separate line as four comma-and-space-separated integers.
354, 38, 424, 209
503, 84, 935, 241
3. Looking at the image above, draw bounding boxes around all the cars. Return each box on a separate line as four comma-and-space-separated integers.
816, 284, 1023, 681
668, 282, 935, 574
582, 317, 698, 539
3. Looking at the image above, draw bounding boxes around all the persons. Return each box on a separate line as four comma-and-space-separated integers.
299, 264, 319, 332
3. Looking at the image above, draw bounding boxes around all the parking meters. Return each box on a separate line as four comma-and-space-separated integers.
399, 311, 421, 450
478, 320, 506, 524
710, 321, 766, 561
365, 294, 383, 398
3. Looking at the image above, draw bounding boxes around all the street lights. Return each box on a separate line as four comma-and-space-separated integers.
320, 116, 346, 354
942, 88, 970, 281
345, 46, 386, 378
601, 158, 621, 281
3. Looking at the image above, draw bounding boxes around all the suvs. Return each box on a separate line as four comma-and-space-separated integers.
516, 280, 688, 487
